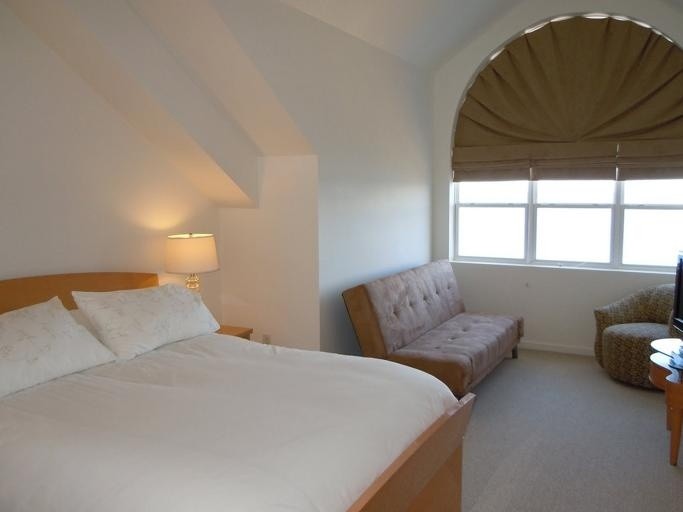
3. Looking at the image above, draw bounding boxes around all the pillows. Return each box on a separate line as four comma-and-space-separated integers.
0, 282, 220, 400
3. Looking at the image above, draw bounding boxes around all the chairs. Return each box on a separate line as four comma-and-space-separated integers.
592, 282, 675, 390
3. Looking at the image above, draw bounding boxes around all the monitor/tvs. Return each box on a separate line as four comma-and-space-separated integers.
673, 259, 683, 333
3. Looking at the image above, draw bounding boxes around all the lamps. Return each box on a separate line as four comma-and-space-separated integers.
165, 232, 221, 293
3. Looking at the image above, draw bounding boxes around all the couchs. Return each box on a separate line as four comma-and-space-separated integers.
341, 259, 524, 400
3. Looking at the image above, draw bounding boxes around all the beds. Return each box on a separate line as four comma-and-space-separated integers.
0, 272, 477, 512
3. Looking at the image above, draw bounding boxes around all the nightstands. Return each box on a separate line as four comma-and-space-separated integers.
215, 326, 252, 341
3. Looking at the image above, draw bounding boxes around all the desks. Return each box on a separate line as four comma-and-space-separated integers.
648, 335, 682, 466
